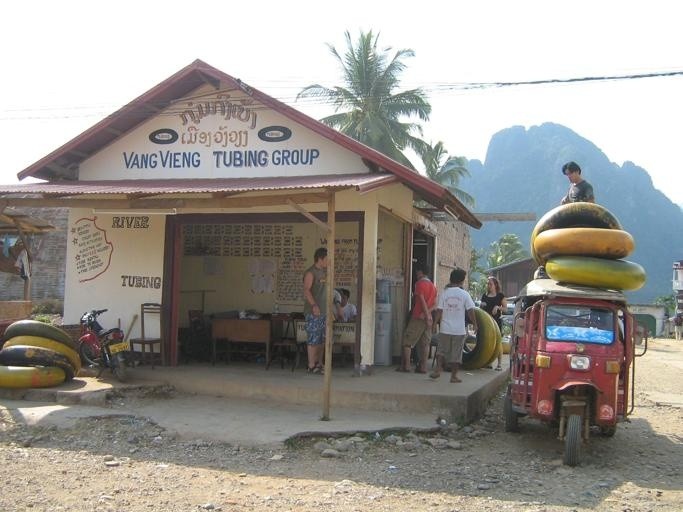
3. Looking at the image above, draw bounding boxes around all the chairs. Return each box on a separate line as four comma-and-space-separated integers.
413, 310, 440, 367
266, 318, 306, 371
130, 303, 167, 370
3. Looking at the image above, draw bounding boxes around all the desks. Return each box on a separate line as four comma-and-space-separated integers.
211, 318, 276, 370
291, 322, 359, 372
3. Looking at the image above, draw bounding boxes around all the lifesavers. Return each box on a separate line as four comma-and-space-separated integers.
0, 319, 81, 389
530, 201, 646, 291
461, 307, 510, 370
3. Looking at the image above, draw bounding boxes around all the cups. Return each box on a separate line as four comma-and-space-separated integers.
239, 312, 248, 317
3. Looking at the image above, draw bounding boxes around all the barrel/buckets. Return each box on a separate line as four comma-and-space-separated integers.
376, 279, 390, 303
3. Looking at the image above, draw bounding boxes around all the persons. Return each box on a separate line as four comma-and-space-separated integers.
480, 276, 508, 371
301, 248, 326, 376
337, 288, 358, 323
429, 267, 477, 382
331, 288, 343, 348
394, 261, 438, 373
559, 161, 594, 205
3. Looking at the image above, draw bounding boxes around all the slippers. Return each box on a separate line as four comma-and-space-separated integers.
393, 364, 410, 373
413, 367, 427, 374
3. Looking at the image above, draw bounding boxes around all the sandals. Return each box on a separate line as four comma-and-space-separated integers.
305, 361, 325, 376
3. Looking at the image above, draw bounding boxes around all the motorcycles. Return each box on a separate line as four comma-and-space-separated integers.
502, 299, 649, 467
80, 307, 129, 384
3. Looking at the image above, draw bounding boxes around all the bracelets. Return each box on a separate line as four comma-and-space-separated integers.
311, 303, 319, 308
